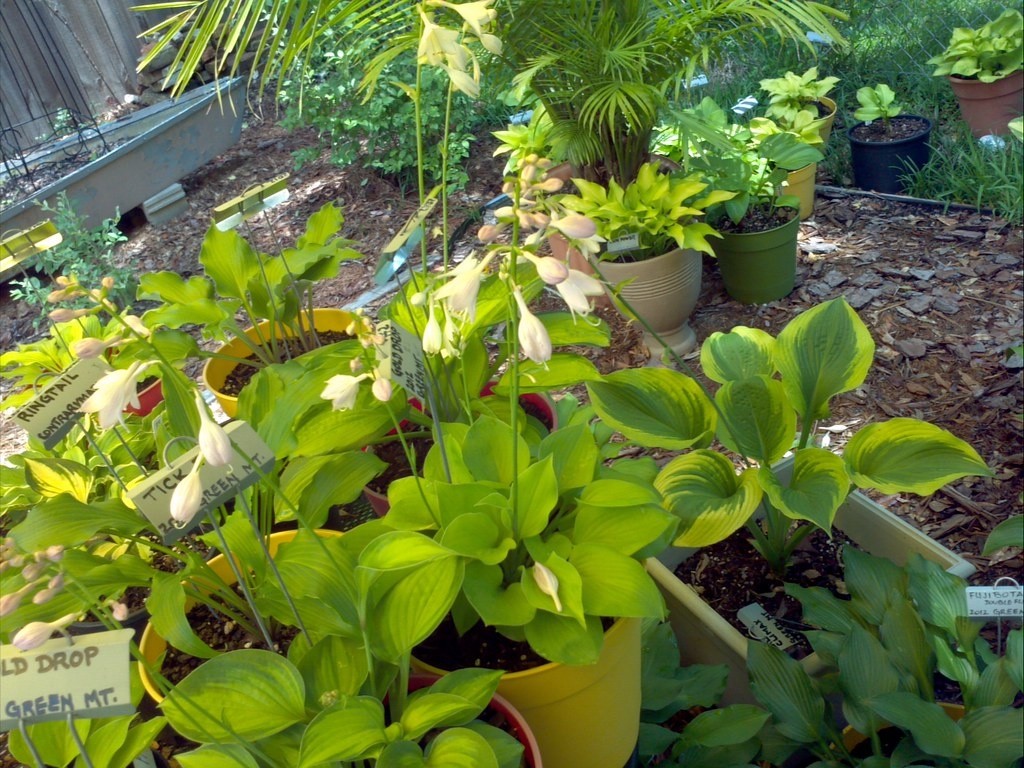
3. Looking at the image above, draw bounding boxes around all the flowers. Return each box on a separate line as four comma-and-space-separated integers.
0, 0, 688, 768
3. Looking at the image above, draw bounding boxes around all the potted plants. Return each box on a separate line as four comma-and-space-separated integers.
925, 8, 1024, 140
128, 0, 844, 374
585, 292, 1024, 767
845, 84, 933, 196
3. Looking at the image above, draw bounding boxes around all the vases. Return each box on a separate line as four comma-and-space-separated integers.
205, 307, 366, 420
407, 561, 644, 767
359, 383, 558, 518
135, 528, 346, 703
